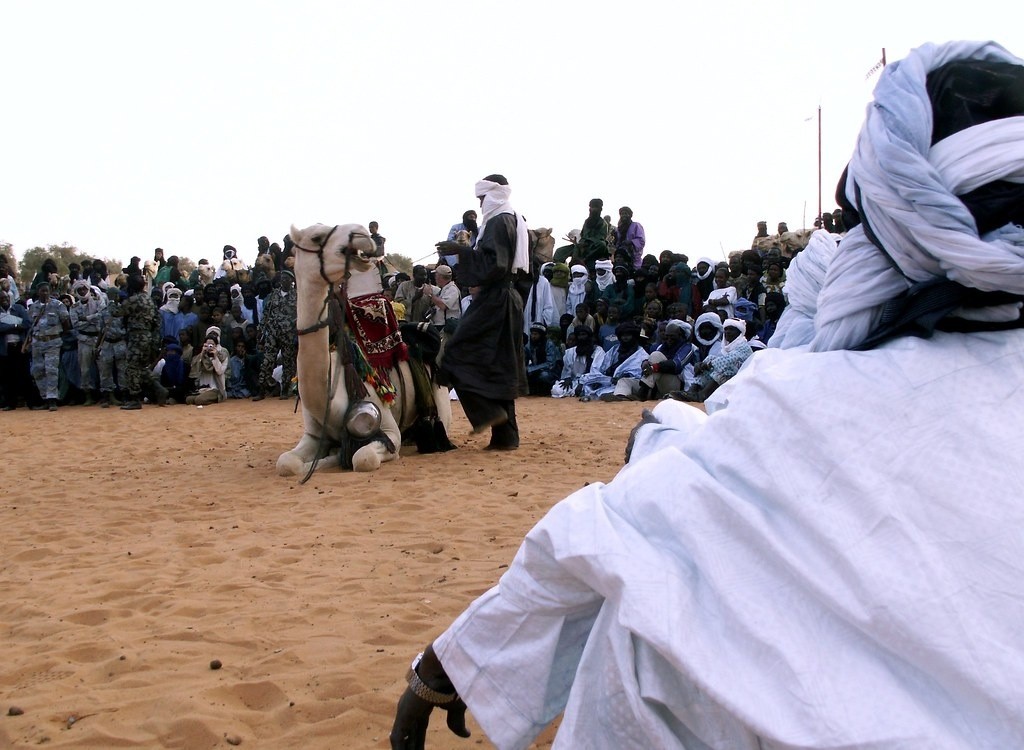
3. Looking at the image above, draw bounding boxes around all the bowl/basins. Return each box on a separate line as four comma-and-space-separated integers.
343, 401, 382, 442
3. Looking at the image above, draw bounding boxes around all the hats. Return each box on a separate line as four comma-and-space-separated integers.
431, 265, 453, 276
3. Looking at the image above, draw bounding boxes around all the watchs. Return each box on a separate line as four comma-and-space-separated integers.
405, 652, 460, 703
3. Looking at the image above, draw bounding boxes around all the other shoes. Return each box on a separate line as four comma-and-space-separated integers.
0, 394, 176, 414
483, 444, 518, 451
474, 409, 507, 433
628, 393, 644, 402
670, 389, 700, 401
280, 393, 288, 400
601, 392, 623, 401
252, 392, 265, 401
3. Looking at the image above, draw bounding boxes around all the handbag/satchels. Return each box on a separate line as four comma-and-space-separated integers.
444, 317, 461, 334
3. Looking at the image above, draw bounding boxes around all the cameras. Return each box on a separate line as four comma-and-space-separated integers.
207, 343, 214, 350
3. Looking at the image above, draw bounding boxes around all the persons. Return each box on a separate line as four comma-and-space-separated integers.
525, 199, 845, 463
0, 233, 298, 410
360, 210, 480, 401
389, 39, 1024, 750
434, 175, 532, 449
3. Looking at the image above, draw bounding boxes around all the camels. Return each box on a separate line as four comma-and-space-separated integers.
274, 222, 454, 478
